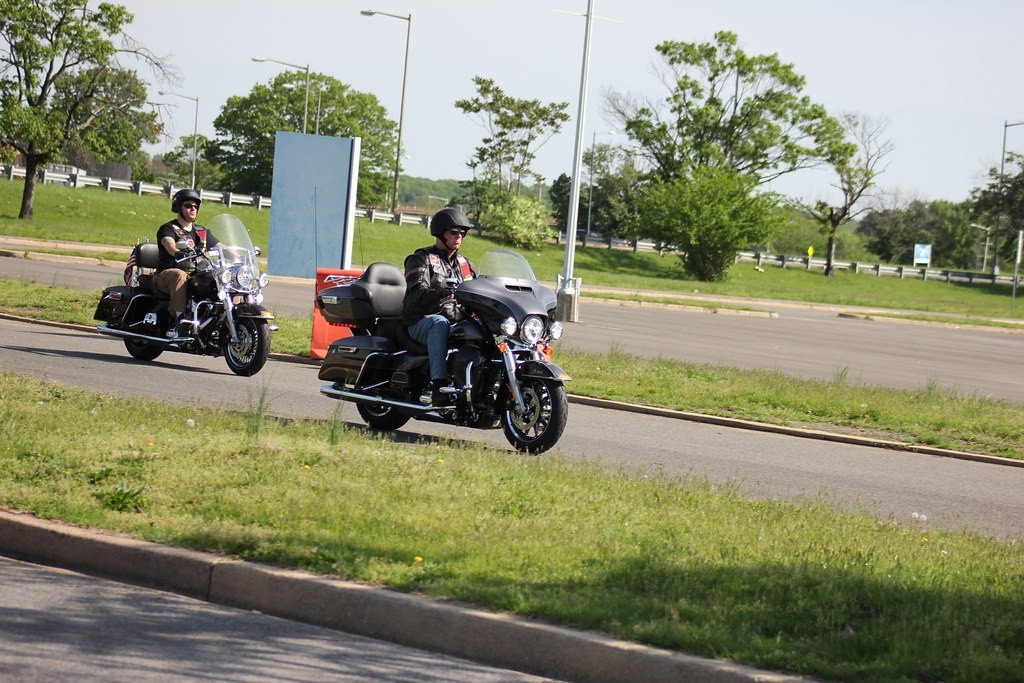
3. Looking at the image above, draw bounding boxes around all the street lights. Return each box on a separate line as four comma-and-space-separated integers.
158, 91, 198, 189
586, 130, 613, 237
251, 56, 309, 135
971, 222, 991, 272
360, 10, 413, 216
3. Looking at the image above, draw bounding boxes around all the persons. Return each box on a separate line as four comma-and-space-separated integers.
403, 209, 480, 408
154, 189, 254, 338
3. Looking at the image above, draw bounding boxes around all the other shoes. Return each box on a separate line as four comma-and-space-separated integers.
432, 379, 451, 406
176, 312, 193, 335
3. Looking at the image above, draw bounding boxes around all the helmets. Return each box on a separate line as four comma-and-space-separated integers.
431, 209, 472, 238
172, 189, 201, 212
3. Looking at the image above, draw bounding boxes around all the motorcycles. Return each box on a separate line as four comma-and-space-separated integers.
92, 213, 278, 376
318, 249, 573, 454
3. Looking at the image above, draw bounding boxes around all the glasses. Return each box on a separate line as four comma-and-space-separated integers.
180, 203, 199, 210
446, 229, 467, 236
